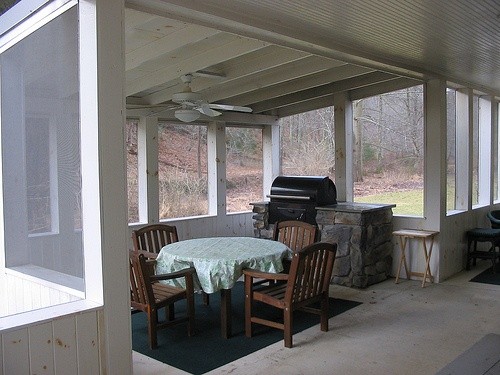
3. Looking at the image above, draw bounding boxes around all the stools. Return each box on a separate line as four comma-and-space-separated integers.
467, 229, 500, 269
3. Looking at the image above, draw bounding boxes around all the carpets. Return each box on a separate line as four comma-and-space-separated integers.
130, 281, 364, 375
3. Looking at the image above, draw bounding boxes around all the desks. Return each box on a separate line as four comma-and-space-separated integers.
153, 237, 295, 339
392, 227, 439, 288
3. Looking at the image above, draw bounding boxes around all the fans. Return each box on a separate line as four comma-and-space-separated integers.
151, 73, 252, 118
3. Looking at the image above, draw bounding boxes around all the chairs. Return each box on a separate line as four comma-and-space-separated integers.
129, 248, 196, 350
131, 224, 179, 260
487, 209, 500, 252
272, 221, 318, 278
242, 242, 338, 347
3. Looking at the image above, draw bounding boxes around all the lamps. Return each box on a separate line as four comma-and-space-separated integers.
172, 73, 202, 123
